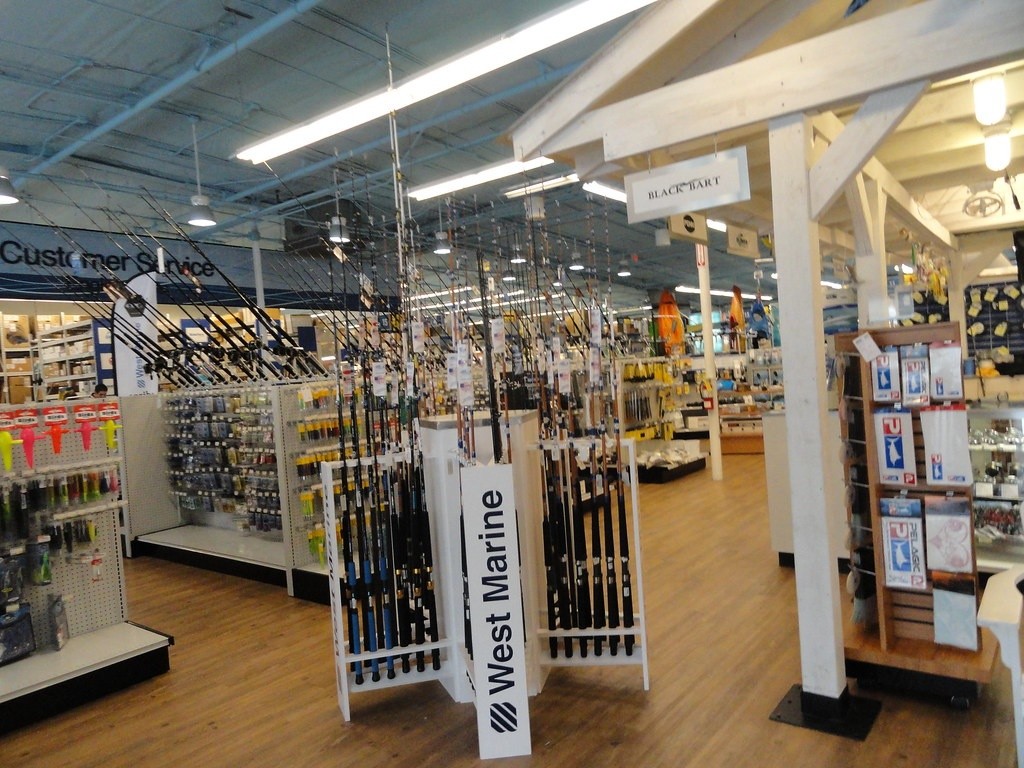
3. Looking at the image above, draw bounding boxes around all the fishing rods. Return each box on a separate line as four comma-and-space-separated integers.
1, 162, 329, 387
329, 20, 660, 686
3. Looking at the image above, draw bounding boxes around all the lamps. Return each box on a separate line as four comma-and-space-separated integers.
0, 114, 633, 286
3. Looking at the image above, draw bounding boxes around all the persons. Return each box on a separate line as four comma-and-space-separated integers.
87, 383, 108, 400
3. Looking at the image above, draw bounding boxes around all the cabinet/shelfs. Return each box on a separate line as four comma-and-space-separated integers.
1, 309, 651, 405
